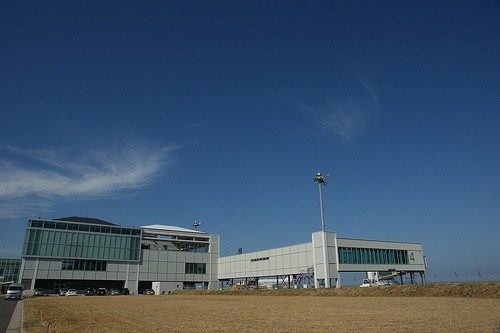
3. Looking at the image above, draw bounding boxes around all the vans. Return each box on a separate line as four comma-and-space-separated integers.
5, 283, 25, 300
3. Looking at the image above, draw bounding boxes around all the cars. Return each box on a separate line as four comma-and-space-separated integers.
85, 288, 129, 296
143, 289, 155, 295
33, 288, 45, 296
59, 288, 78, 296
